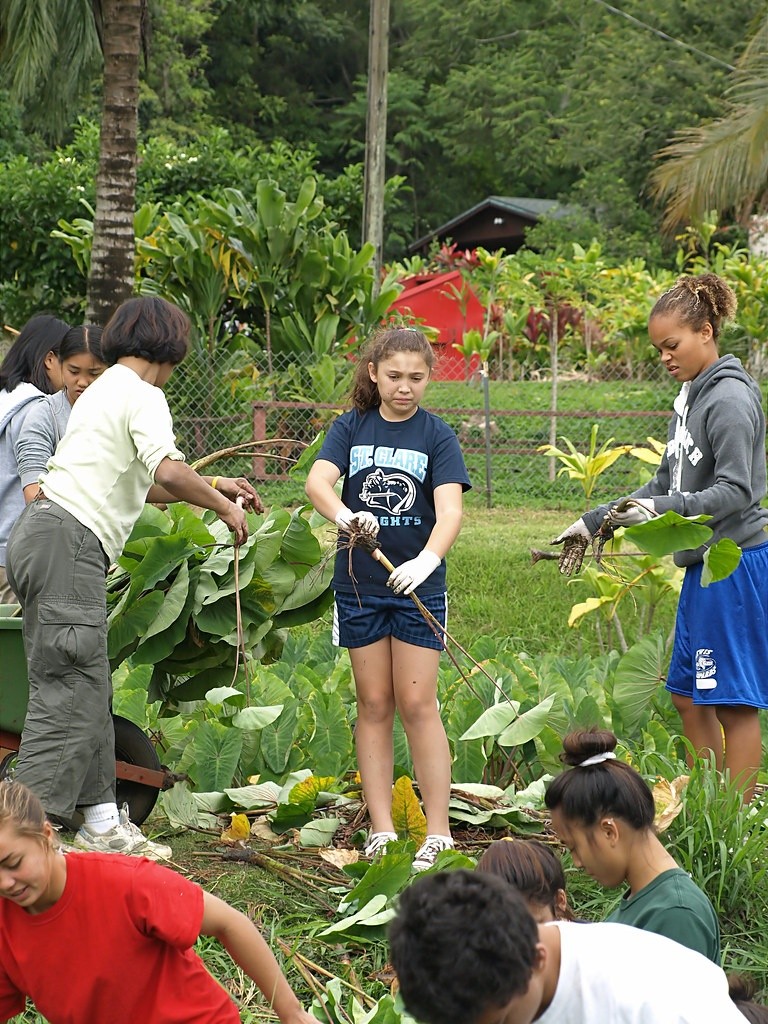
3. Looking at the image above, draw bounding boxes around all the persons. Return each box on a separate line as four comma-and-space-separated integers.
0, 308, 75, 605
551, 275, 767, 811
13, 324, 111, 505
387, 869, 750, 1024
470, 838, 590, 926
0, 774, 319, 1024
545, 726, 721, 967
306, 328, 472, 870
11, 295, 263, 864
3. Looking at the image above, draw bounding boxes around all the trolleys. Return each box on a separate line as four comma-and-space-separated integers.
0, 600, 175, 837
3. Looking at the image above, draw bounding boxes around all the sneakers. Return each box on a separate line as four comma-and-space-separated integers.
410, 834, 456, 874
73, 802, 172, 861
364, 832, 399, 862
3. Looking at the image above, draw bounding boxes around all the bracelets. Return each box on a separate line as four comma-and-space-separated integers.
211, 475, 221, 488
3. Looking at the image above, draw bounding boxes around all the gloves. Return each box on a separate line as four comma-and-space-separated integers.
548, 517, 592, 577
335, 508, 380, 539
386, 549, 441, 596
604, 497, 654, 528
